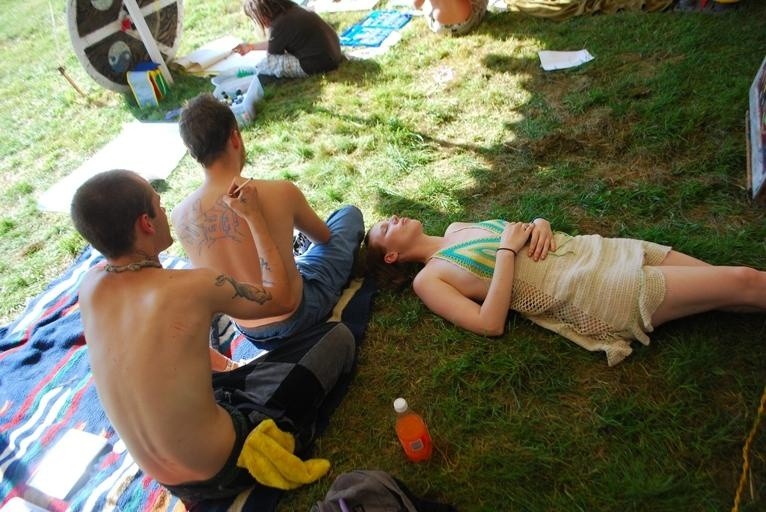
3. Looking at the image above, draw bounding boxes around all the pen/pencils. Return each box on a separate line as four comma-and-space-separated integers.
230, 177, 254, 197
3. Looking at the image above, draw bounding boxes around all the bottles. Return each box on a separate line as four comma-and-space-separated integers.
393, 397, 433, 462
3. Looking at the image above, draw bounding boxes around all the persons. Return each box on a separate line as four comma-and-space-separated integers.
232, 1, 342, 76
70, 170, 356, 504
414, 0, 489, 38
170, 94, 364, 347
364, 216, 766, 366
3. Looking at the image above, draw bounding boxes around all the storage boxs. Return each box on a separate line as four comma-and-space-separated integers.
212, 76, 264, 124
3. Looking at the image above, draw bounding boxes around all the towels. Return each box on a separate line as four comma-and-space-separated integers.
236, 419, 335, 497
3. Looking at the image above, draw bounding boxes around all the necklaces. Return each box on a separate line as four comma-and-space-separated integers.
103, 261, 164, 273
